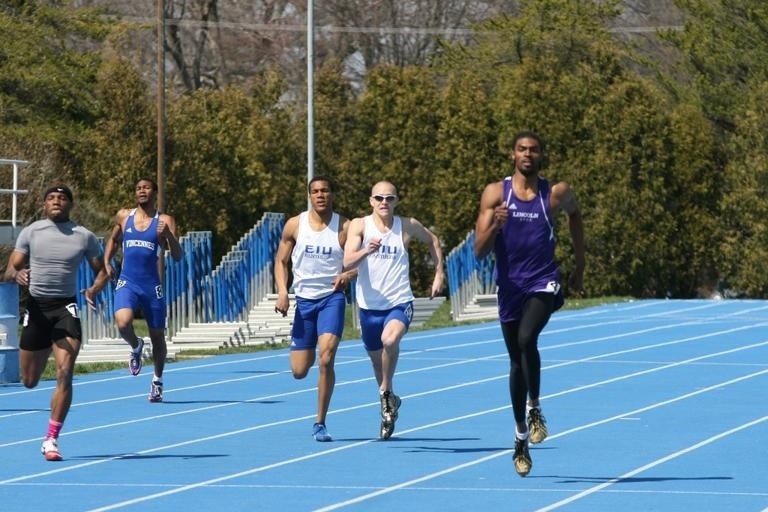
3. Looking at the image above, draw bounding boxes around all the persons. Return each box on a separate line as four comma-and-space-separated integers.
274, 177, 359, 440
343, 181, 445, 439
5, 183, 110, 462
474, 131, 585, 475
103, 178, 181, 403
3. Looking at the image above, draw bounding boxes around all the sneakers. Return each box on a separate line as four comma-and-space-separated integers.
41, 439, 62, 461
512, 435, 531, 478
314, 424, 332, 442
380, 397, 402, 439
527, 408, 548, 445
148, 380, 163, 401
129, 339, 144, 375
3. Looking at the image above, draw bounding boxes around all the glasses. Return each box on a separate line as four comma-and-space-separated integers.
370, 195, 397, 204
